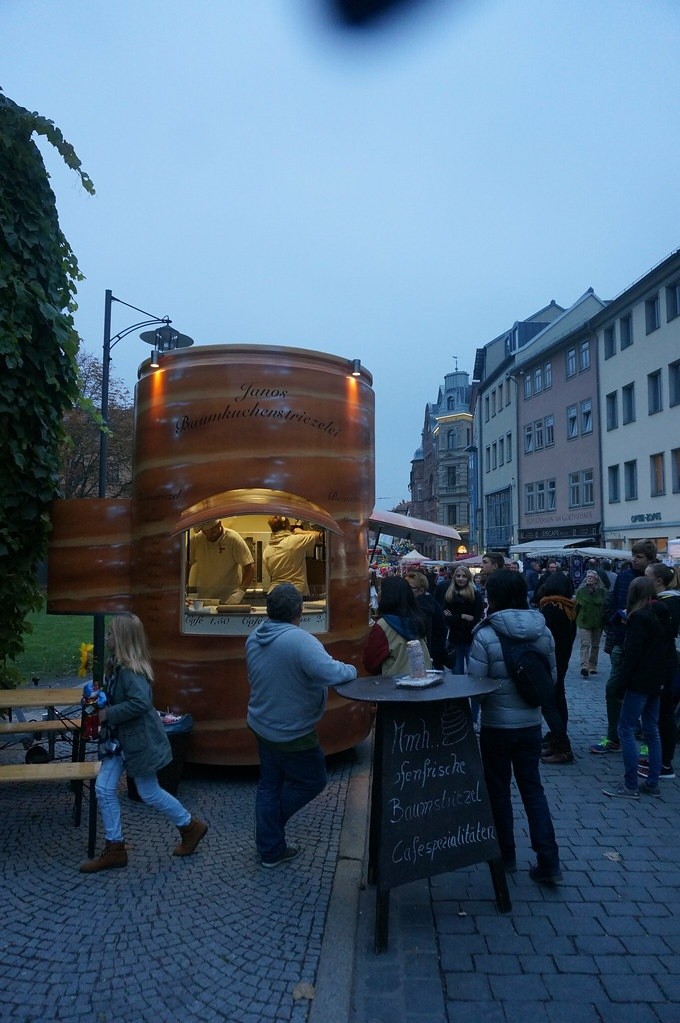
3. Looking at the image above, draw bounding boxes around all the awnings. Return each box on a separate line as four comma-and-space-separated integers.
509, 538, 592, 553
368, 510, 462, 563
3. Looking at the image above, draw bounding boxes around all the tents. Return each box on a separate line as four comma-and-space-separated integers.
401, 549, 430, 561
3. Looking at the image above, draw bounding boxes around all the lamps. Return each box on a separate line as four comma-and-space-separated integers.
150, 350, 165, 368
348, 358, 361, 376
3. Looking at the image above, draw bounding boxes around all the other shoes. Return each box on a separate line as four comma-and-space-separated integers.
590, 671, 597, 674
542, 745, 557, 757
530, 864, 564, 881
541, 751, 574, 764
581, 669, 588, 677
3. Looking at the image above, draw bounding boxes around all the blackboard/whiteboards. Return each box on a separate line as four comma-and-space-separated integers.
361, 701, 502, 888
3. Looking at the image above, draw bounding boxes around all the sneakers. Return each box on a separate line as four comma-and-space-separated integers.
80, 840, 128, 873
602, 783, 640, 799
262, 843, 302, 868
637, 764, 675, 778
588, 736, 622, 753
639, 779, 661, 796
173, 817, 209, 856
638, 744, 649, 755
638, 758, 651, 769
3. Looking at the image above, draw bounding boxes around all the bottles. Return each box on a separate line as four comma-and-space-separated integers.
81, 682, 99, 741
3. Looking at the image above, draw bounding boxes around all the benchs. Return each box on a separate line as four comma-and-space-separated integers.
0, 717, 83, 763
0, 760, 103, 861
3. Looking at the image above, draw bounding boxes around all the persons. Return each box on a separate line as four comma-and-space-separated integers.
362, 577, 432, 676
370, 552, 633, 748
539, 570, 578, 763
601, 577, 672, 799
188, 520, 255, 605
80, 613, 208, 873
244, 584, 358, 867
635, 563, 680, 779
589, 539, 657, 753
262, 515, 322, 601
468, 571, 564, 882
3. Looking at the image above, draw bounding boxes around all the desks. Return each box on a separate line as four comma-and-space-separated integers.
0, 689, 85, 794
335, 673, 512, 960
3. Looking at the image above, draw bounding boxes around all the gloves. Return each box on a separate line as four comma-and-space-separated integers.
225, 588, 245, 605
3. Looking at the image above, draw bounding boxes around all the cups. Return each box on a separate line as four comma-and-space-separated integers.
194, 600, 203, 609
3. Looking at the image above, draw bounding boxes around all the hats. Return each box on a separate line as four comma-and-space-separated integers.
193, 520, 220, 533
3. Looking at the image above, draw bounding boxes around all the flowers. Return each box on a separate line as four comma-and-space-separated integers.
71, 641, 98, 679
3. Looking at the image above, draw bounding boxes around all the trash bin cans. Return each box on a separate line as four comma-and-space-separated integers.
126, 712, 192, 802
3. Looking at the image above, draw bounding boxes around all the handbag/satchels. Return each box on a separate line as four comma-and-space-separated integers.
494, 629, 555, 707
